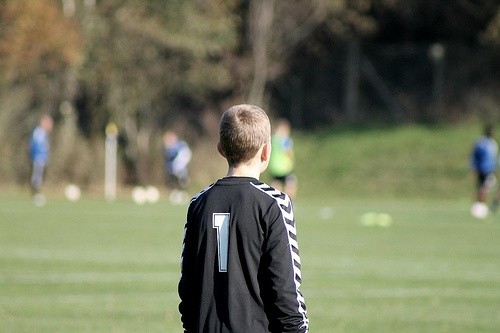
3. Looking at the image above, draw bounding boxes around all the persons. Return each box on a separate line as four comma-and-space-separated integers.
469, 122, 498, 219
176, 105, 309, 333
28, 115, 295, 206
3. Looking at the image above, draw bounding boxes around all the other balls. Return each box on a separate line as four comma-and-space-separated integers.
65, 183, 82, 200
469, 200, 487, 219
